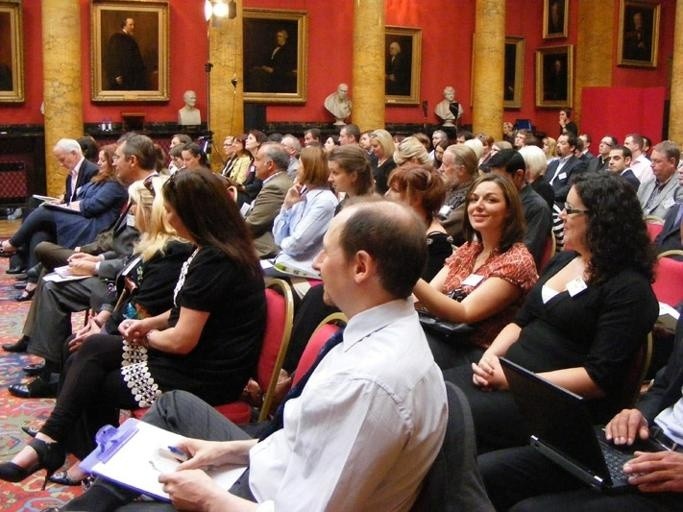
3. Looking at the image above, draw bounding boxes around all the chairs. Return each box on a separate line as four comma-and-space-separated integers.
131, 277, 295, 432
650, 248, 682, 308
534, 231, 553, 272
290, 311, 348, 395
640, 213, 663, 244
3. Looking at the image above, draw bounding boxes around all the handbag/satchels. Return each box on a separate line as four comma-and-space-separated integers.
418, 287, 480, 344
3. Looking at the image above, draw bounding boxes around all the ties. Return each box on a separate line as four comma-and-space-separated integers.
257, 327, 343, 441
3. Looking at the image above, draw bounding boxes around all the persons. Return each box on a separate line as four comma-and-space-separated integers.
559, 108, 577, 138
177, 90, 202, 127
323, 81, 352, 127
433, 85, 466, 129
505, 48, 515, 101
548, 1, 564, 33
384, 42, 407, 97
244, 29, 292, 93
45, 198, 448, 511
624, 9, 652, 62
543, 60, 566, 102
221, 123, 683, 512
0, 168, 268, 493
0, 132, 211, 437
105, 17, 151, 91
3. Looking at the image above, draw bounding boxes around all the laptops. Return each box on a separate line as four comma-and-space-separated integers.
497, 356, 668, 498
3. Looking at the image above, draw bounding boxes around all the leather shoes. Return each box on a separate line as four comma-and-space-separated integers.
22, 361, 44, 373
7, 382, 56, 398
2, 339, 28, 354
0, 240, 42, 302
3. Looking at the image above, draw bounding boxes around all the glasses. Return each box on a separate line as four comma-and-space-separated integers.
600, 140, 613, 147
168, 166, 187, 211
564, 201, 592, 217
143, 173, 159, 198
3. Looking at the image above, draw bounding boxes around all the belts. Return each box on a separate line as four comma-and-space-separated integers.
649, 421, 683, 452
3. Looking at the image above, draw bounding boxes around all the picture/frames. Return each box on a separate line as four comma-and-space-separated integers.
535, 43, 573, 110
385, 25, 421, 105
470, 32, 525, 110
543, 0, 569, 39
0, 0, 26, 104
90, 1, 170, 104
243, 8, 307, 104
616, 0, 661, 69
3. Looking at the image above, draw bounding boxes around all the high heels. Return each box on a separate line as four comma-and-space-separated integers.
49, 461, 87, 487
0, 425, 65, 492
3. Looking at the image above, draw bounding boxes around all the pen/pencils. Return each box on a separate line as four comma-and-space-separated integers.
168, 445, 191, 460
